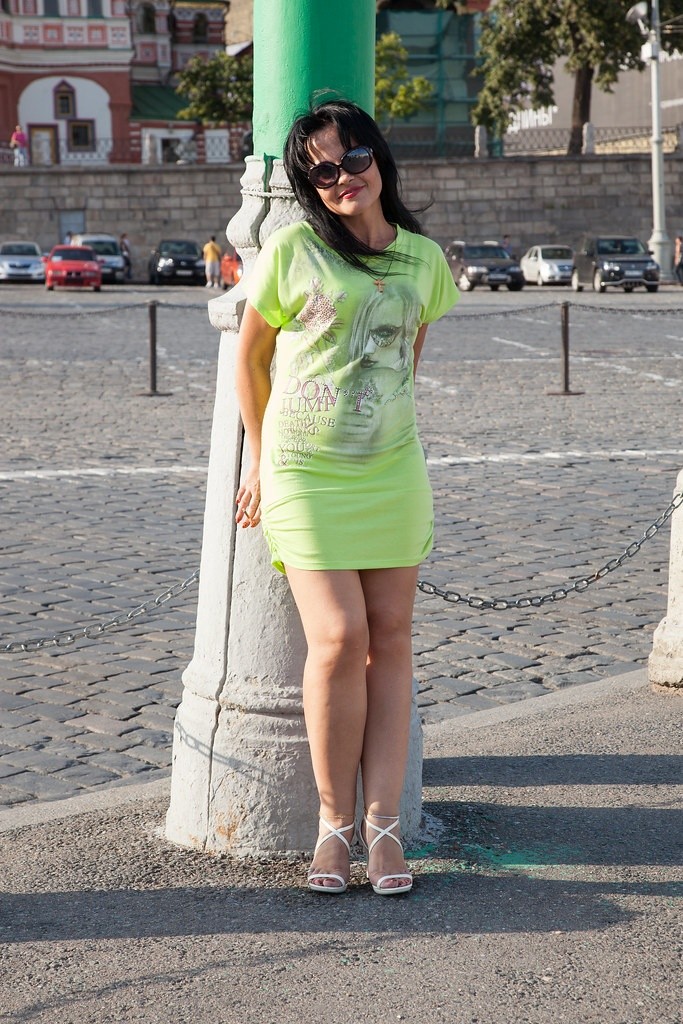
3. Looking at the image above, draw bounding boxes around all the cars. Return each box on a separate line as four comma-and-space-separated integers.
443, 240, 525, 291
220, 244, 245, 288
519, 245, 577, 286
41, 244, 106, 292
0, 239, 45, 282
146, 238, 209, 286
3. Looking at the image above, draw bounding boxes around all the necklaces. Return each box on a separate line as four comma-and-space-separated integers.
343, 225, 398, 292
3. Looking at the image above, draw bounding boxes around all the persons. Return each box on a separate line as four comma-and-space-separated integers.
674, 236, 683, 286
121, 234, 132, 279
64, 231, 73, 245
502, 234, 512, 252
203, 236, 223, 288
232, 104, 459, 895
11, 125, 27, 166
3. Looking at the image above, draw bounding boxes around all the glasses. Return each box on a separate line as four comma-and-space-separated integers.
307, 144, 373, 189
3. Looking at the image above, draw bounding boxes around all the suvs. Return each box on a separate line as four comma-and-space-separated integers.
569, 232, 660, 293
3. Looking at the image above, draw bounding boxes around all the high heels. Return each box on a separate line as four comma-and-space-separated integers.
307, 807, 413, 894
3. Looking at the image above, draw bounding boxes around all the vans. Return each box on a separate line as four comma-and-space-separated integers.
70, 233, 125, 283
